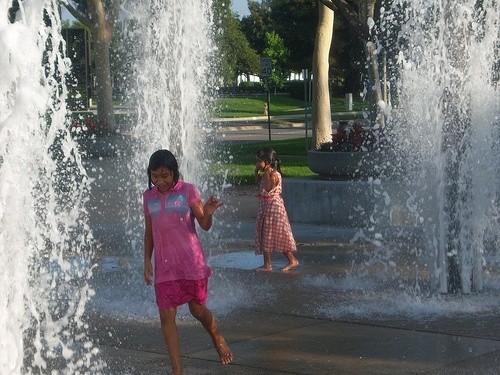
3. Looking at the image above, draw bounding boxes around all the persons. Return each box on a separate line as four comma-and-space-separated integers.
252, 147, 299, 272
140, 148, 235, 375
